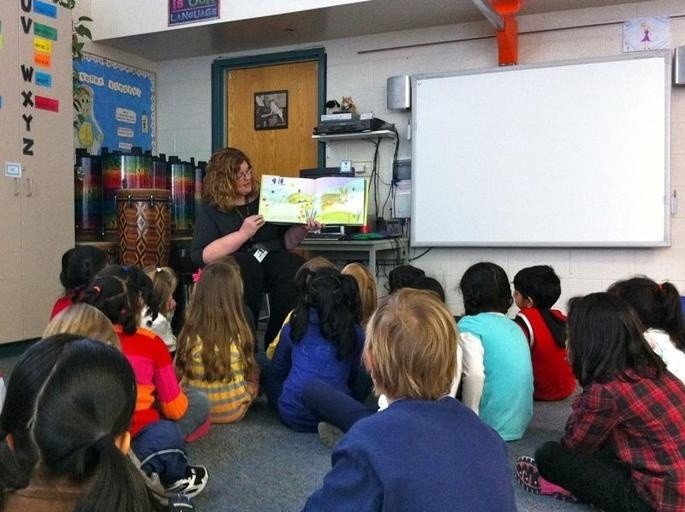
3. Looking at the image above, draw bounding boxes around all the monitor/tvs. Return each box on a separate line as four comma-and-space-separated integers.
300, 167, 359, 235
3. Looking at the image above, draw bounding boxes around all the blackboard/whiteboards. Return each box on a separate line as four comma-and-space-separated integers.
409, 49, 673, 248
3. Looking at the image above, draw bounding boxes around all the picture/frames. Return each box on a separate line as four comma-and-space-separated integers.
253, 89, 287, 129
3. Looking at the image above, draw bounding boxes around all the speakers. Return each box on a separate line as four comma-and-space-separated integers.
387, 75, 411, 110
675, 46, 685, 85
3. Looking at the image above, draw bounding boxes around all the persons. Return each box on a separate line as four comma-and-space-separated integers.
190, 147, 325, 352
262, 257, 685, 511
1, 245, 262, 512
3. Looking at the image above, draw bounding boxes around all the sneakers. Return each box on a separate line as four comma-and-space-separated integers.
165, 465, 208, 499
515, 456, 582, 504
318, 422, 344, 449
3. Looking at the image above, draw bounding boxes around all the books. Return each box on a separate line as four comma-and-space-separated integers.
256, 173, 370, 227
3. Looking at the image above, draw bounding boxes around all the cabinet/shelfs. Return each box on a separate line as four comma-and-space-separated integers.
1, 1, 74, 346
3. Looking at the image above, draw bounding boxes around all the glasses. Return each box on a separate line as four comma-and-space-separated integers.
239, 166, 253, 181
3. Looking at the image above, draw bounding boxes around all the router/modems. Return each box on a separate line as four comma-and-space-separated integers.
320, 113, 352, 122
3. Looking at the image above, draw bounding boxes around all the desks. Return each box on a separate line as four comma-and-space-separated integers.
296, 233, 409, 286
